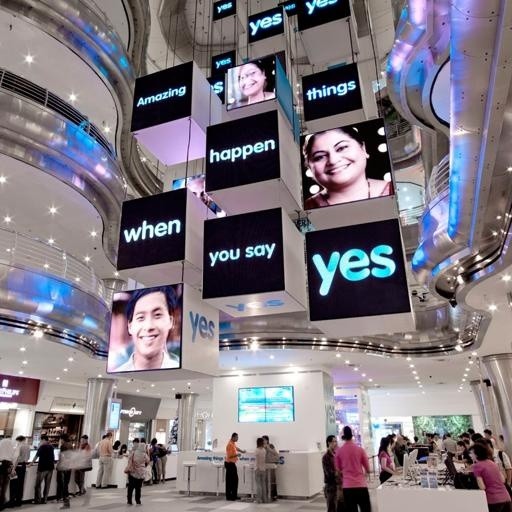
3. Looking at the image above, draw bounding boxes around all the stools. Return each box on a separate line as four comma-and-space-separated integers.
181, 463, 196, 498
243, 463, 260, 501
263, 464, 278, 503
210, 462, 225, 500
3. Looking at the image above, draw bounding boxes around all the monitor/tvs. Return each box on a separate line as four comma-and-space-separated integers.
27, 449, 40, 463
416, 444, 433, 452
408, 447, 429, 458
104, 398, 122, 431
54, 447, 61, 461
403, 454, 417, 486
409, 449, 422, 475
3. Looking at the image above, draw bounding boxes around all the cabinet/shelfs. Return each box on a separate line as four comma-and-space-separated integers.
375, 462, 490, 512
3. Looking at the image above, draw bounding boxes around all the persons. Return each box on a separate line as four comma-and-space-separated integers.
322, 435, 344, 512
181, 175, 213, 209
333, 425, 372, 512
224, 431, 246, 501
115, 285, 179, 371
1, 429, 173, 507
411, 289, 424, 304
376, 426, 512, 512
260, 434, 280, 502
229, 61, 276, 109
244, 438, 270, 504
299, 127, 394, 210
